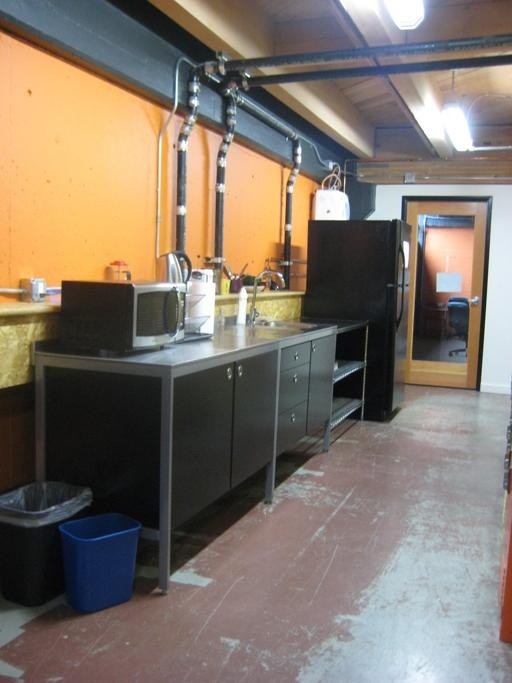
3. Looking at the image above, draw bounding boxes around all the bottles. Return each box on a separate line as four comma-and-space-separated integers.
236, 286, 248, 325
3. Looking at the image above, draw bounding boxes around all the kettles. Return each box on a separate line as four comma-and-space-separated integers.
158, 251, 192, 286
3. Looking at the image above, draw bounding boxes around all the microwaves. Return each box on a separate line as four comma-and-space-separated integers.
61, 280, 186, 353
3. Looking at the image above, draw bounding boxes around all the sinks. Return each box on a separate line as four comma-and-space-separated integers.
258, 321, 335, 332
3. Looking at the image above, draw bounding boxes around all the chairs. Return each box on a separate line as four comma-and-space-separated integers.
446, 295, 471, 356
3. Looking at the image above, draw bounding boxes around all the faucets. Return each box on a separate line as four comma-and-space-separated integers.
250, 269, 285, 324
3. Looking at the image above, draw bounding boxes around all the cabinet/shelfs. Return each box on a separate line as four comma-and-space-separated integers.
323, 325, 371, 453
277, 335, 336, 456
47, 349, 281, 599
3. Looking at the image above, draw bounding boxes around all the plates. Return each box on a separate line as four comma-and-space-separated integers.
244, 285, 264, 293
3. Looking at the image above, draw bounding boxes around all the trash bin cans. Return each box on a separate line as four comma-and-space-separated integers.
2, 481, 142, 613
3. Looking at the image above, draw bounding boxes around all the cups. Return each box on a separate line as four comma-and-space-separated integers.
221, 278, 242, 294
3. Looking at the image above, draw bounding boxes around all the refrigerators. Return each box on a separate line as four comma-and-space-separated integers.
304, 218, 412, 423
